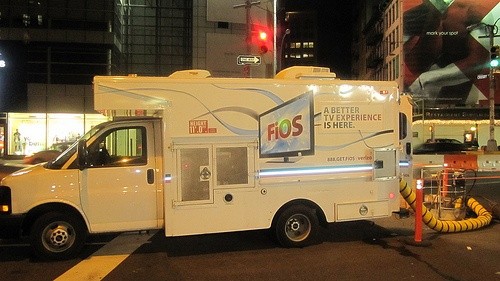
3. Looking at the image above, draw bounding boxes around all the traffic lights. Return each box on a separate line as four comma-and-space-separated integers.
257, 24, 271, 55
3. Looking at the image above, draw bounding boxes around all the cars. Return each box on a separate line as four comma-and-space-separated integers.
24, 150, 65, 163
418, 138, 468, 153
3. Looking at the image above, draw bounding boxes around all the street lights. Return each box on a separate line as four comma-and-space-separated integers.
466, 17, 500, 153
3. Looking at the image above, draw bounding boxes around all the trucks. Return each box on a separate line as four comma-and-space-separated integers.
0, 66, 414, 259
414, 119, 500, 152
